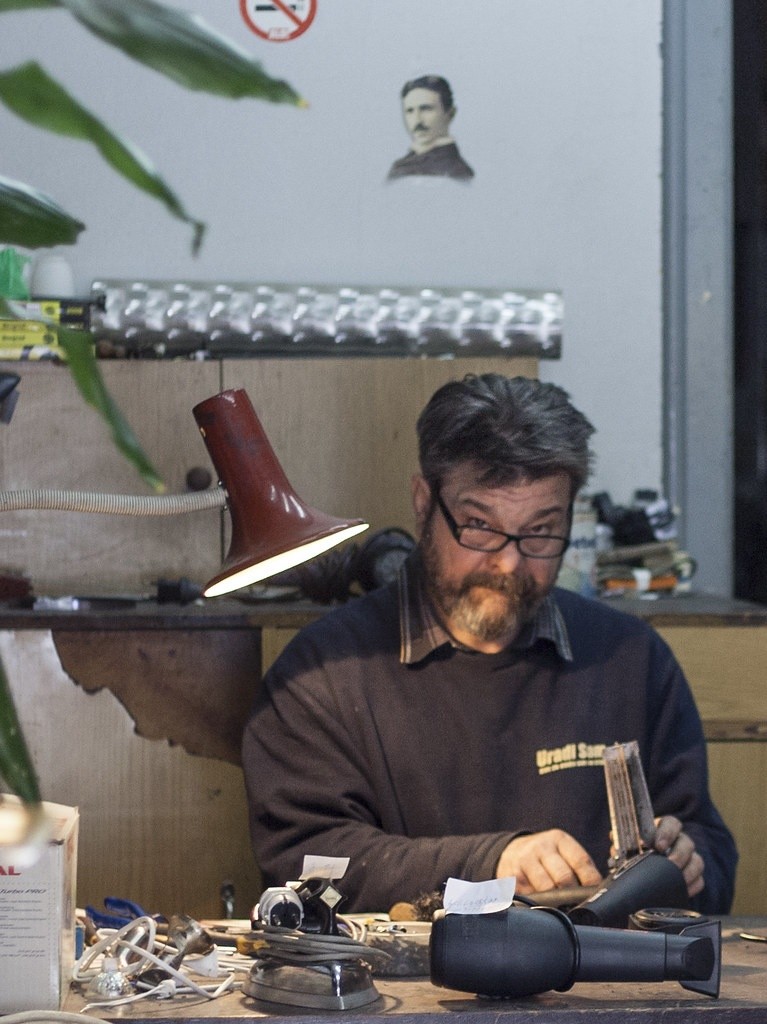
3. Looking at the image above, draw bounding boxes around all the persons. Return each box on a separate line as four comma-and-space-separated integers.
387, 74, 473, 181
240, 371, 739, 914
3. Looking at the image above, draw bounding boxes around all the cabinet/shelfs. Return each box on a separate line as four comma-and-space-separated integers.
0, 389, 767, 920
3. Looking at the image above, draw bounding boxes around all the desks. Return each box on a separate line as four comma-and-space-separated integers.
62, 919, 767, 1024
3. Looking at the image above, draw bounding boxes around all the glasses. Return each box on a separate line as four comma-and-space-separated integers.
436, 492, 572, 559
